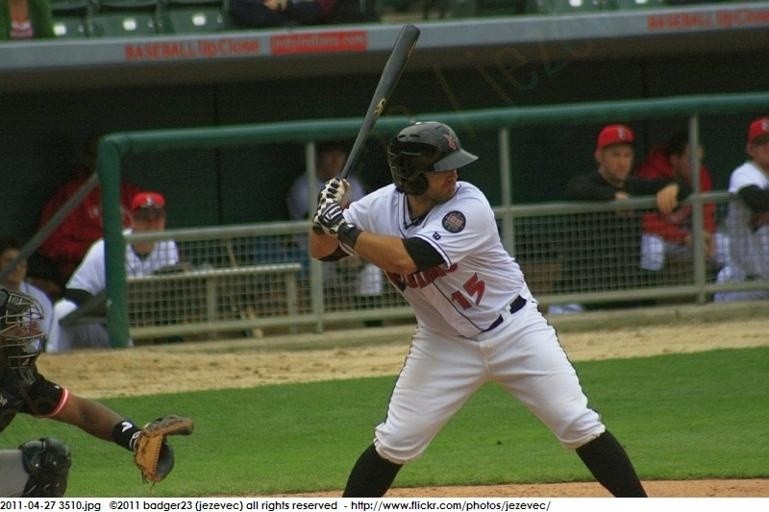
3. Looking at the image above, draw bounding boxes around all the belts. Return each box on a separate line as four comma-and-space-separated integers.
482, 295, 526, 332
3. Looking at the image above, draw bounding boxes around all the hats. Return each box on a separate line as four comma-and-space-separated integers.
749, 117, 769, 144
131, 192, 167, 217
597, 125, 635, 150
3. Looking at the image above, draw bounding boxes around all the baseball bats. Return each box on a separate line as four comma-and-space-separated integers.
312, 23, 420, 233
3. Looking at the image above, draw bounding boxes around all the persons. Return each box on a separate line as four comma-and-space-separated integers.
287, 142, 382, 327
713, 115, 769, 307
307, 121, 647, 496
544, 125, 695, 313
62, 190, 187, 345
228, 1, 328, 28
40, 129, 142, 290
637, 134, 729, 279
1, 248, 133, 353
0, 284, 195, 497
2, 1, 57, 42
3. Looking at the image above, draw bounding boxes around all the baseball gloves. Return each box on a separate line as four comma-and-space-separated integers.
133, 415, 193, 483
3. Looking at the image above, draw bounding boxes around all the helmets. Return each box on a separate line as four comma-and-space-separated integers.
0, 286, 48, 383
387, 121, 480, 195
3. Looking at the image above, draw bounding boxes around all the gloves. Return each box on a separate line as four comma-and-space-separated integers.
318, 177, 351, 208
312, 198, 356, 240
